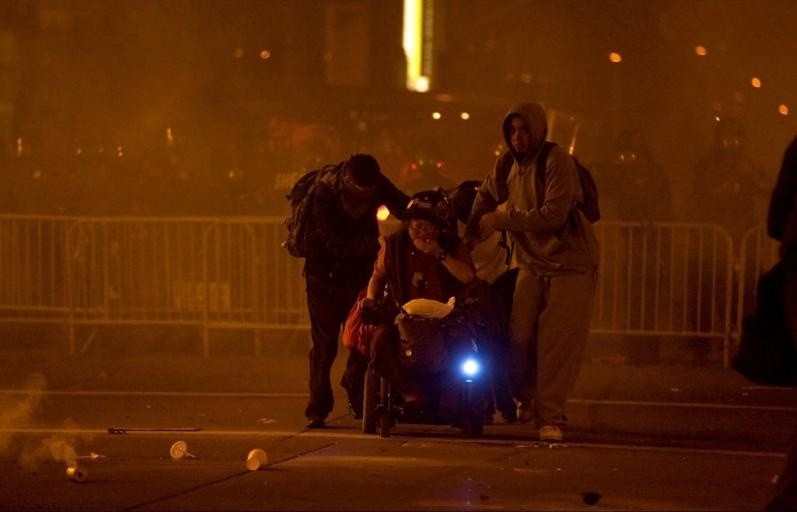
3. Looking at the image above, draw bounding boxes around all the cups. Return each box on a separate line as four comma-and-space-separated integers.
245, 449, 268, 471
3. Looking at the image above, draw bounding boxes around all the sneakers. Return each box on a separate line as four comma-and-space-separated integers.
303, 391, 567, 443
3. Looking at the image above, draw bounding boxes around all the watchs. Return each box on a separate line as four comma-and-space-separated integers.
438, 251, 448, 262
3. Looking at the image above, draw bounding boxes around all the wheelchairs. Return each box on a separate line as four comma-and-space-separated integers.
364, 299, 492, 435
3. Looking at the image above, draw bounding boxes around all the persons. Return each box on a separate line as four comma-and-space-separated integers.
369, 189, 475, 411
766, 136, 796, 511
301, 152, 412, 427
686, 115, 772, 354
605, 130, 673, 357
463, 102, 601, 442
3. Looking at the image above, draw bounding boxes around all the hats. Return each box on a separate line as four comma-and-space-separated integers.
404, 190, 458, 227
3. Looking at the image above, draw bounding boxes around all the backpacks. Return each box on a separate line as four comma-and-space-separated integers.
279, 168, 323, 256
537, 142, 601, 226
